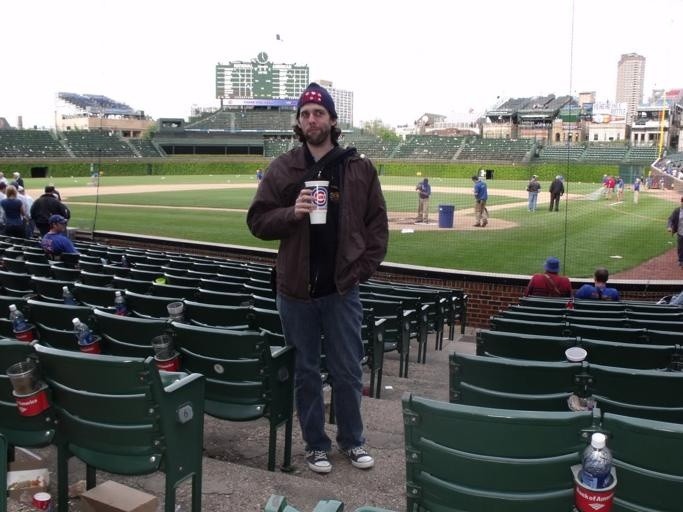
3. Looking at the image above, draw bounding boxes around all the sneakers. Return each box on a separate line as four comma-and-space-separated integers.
415, 220, 429, 224
472, 222, 486, 227
336, 442, 374, 470
303, 446, 333, 474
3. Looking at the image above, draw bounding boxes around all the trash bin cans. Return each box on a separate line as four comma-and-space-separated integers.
439, 205, 454, 228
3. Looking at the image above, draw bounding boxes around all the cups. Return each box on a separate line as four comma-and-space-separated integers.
166, 301, 185, 322
155, 278, 166, 284
151, 334, 174, 359
7, 360, 41, 394
303, 180, 329, 225
30, 491, 54, 511
564, 347, 587, 362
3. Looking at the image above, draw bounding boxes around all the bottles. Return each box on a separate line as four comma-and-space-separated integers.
580, 433, 613, 488
61, 285, 76, 304
71, 317, 93, 344
113, 290, 126, 313
7, 304, 27, 331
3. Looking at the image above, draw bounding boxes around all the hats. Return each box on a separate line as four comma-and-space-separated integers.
296, 82, 338, 119
47, 214, 68, 225
543, 257, 560, 273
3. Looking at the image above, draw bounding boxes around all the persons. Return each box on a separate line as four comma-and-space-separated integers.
523, 257, 572, 298
87, 171, 103, 188
600, 160, 683, 205
667, 196, 682, 267
471, 175, 488, 227
40, 215, 79, 259
547, 174, 564, 210
415, 178, 431, 223
0, 171, 71, 239
479, 170, 486, 177
245, 82, 388, 472
526, 174, 541, 212
573, 267, 619, 301
255, 167, 263, 184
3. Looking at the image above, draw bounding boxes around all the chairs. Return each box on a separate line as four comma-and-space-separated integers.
2, 233, 467, 400
448, 296, 682, 511
2, 296, 297, 474
264, 135, 302, 157
339, 135, 666, 166
2, 129, 166, 161
402, 391, 681, 508
1, 337, 206, 512
183, 109, 296, 134
